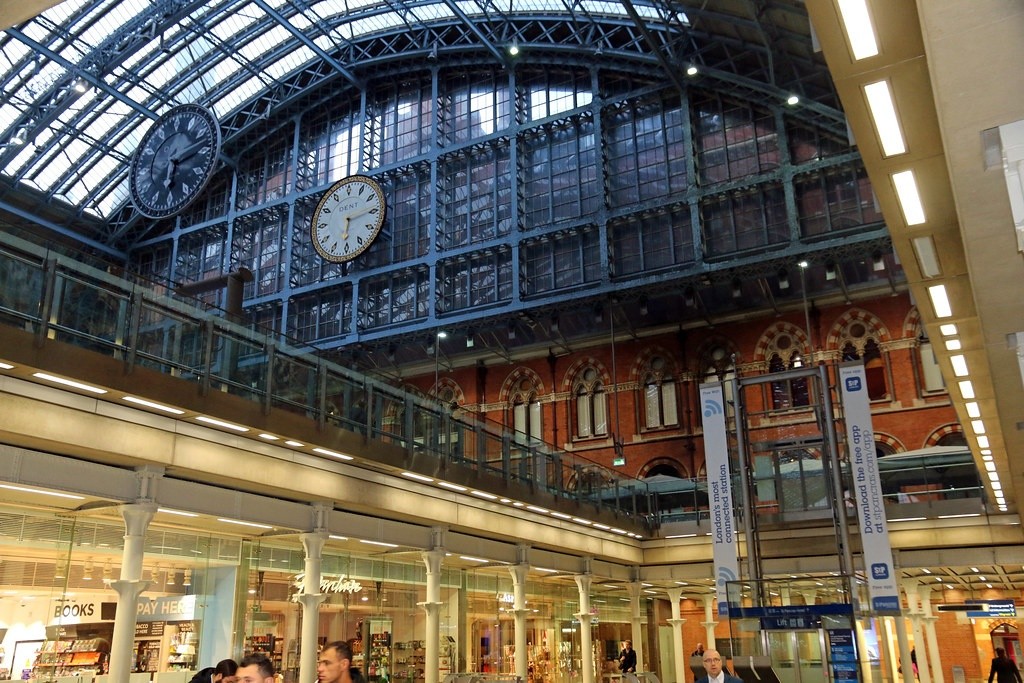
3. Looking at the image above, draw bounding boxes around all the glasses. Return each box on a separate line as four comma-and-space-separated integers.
704, 659, 722, 664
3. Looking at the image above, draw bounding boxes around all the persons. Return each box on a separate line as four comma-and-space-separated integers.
314, 641, 367, 683
696, 649, 743, 683
618, 640, 637, 677
911, 646, 920, 683
988, 647, 1023, 683
691, 643, 704, 683
234, 653, 276, 683
188, 659, 239, 683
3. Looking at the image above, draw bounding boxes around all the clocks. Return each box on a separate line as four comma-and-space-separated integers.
129, 104, 222, 218
310, 175, 387, 264
